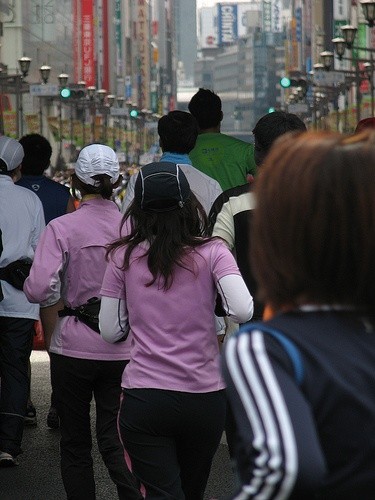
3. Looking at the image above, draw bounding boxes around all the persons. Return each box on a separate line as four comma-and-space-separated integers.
18, 133, 76, 428
26, 141, 149, 500
223, 128, 375, 500
51, 148, 145, 211
122, 109, 233, 262
205, 111, 312, 472
97, 159, 255, 499
355, 117, 375, 135
0, 135, 46, 465
179, 85, 262, 196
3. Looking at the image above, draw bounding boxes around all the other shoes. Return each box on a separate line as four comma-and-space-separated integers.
0, 452, 19, 466
47, 406, 59, 428
25, 406, 38, 426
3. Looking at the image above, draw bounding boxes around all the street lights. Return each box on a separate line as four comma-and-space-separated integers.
285, 25, 375, 127
0, 57, 164, 172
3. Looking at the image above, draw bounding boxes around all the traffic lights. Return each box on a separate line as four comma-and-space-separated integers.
279, 77, 307, 88
268, 108, 282, 113
128, 110, 145, 118
62, 88, 86, 100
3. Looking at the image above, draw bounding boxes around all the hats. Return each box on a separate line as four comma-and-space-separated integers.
134, 161, 191, 213
74, 143, 120, 188
0, 135, 25, 171
